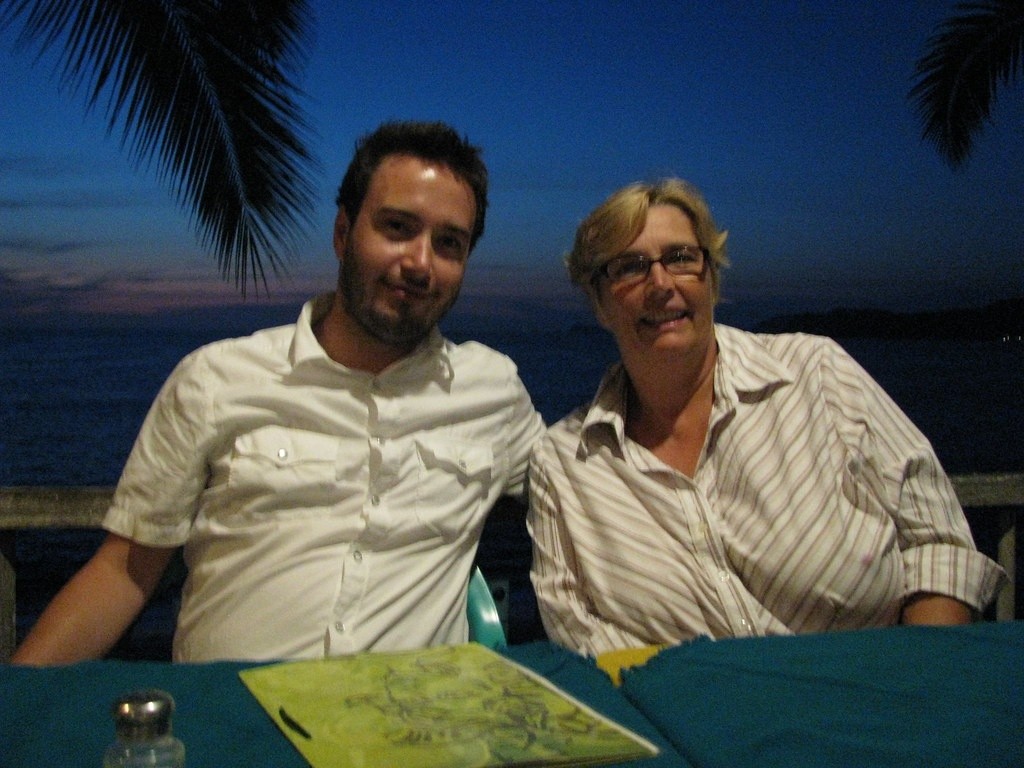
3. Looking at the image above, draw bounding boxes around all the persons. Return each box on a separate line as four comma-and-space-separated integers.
6, 118, 547, 665
526, 178, 1011, 659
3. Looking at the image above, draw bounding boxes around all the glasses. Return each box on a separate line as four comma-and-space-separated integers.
598, 245, 711, 282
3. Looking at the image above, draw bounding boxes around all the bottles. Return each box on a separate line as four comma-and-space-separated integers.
102, 689, 186, 768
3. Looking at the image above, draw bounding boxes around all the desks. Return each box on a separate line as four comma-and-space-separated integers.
586, 620, 1024, 768
0, 639, 693, 768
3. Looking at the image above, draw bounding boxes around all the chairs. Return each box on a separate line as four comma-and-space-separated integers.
129, 561, 506, 665
